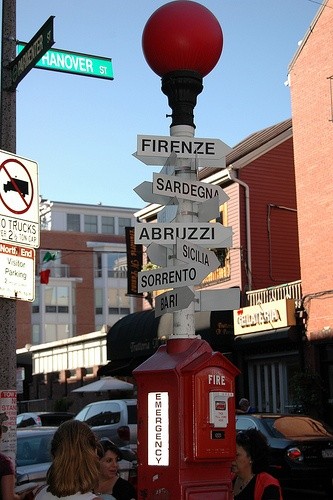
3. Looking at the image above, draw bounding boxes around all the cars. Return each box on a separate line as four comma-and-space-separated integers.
15, 426, 138, 500
234, 413, 333, 491
16, 411, 77, 429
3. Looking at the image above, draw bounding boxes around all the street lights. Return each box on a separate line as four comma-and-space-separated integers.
125, 0, 242, 500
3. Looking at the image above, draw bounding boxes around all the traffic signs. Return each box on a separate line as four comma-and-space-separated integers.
134, 223, 236, 246
153, 171, 230, 208
137, 265, 205, 294
154, 287, 197, 314
175, 237, 222, 272
137, 135, 235, 159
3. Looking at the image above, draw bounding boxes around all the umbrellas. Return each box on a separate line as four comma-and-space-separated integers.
70, 376, 134, 400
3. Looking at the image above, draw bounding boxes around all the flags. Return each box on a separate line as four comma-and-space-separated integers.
43, 251, 55, 263
40, 269, 50, 284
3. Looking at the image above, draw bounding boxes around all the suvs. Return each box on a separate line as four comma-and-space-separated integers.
71, 399, 137, 446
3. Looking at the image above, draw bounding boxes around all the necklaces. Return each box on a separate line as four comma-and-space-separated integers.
97, 493, 103, 495
239, 476, 253, 490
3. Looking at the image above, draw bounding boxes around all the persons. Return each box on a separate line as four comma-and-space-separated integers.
238, 398, 257, 415
32, 420, 103, 500
232, 429, 282, 500
0, 419, 22, 500
91, 439, 137, 500
